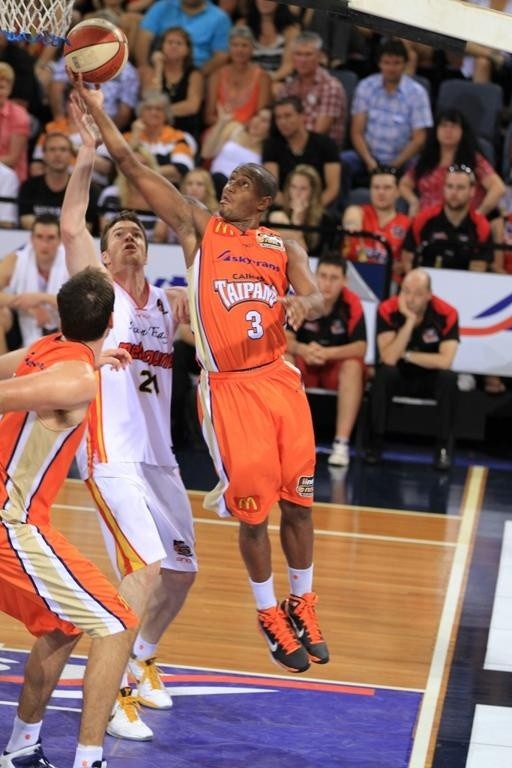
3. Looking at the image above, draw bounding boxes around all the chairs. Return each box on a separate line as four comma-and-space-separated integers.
391, 394, 440, 411
434, 80, 502, 141
326, 68, 358, 150
303, 381, 372, 456
350, 186, 408, 217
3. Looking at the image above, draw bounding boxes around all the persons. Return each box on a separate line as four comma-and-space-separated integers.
170, 166, 216, 244
31, 80, 116, 186
402, 159, 493, 276
262, 96, 343, 211
363, 271, 461, 471
20, 132, 98, 236
1, 156, 19, 228
341, 166, 402, 269
284, 254, 368, 468
135, 0, 232, 77
114, 90, 196, 184
202, 106, 276, 198
234, 0, 303, 100
269, 29, 347, 152
206, 23, 273, 126
82, 1, 141, 56
140, 26, 205, 136
0, 265, 144, 768
451, 0, 511, 83
44, 92, 191, 743
277, 166, 333, 252
0, 63, 31, 181
0, 212, 70, 353
342, 36, 434, 193
401, 107, 507, 273
50, 9, 137, 129
63, 62, 331, 673
97, 140, 169, 241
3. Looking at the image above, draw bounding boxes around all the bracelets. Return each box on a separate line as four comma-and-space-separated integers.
405, 351, 411, 361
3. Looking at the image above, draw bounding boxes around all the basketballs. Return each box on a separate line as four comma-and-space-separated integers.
62, 17, 129, 85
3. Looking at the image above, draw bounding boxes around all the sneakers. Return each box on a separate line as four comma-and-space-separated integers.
128, 654, 174, 710
106, 672, 154, 741
281, 591, 330, 665
256, 600, 311, 674
0, 735, 56, 768
327, 444, 349, 467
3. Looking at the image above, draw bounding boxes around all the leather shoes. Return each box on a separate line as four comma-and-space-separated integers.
364, 442, 380, 465
434, 434, 454, 469
